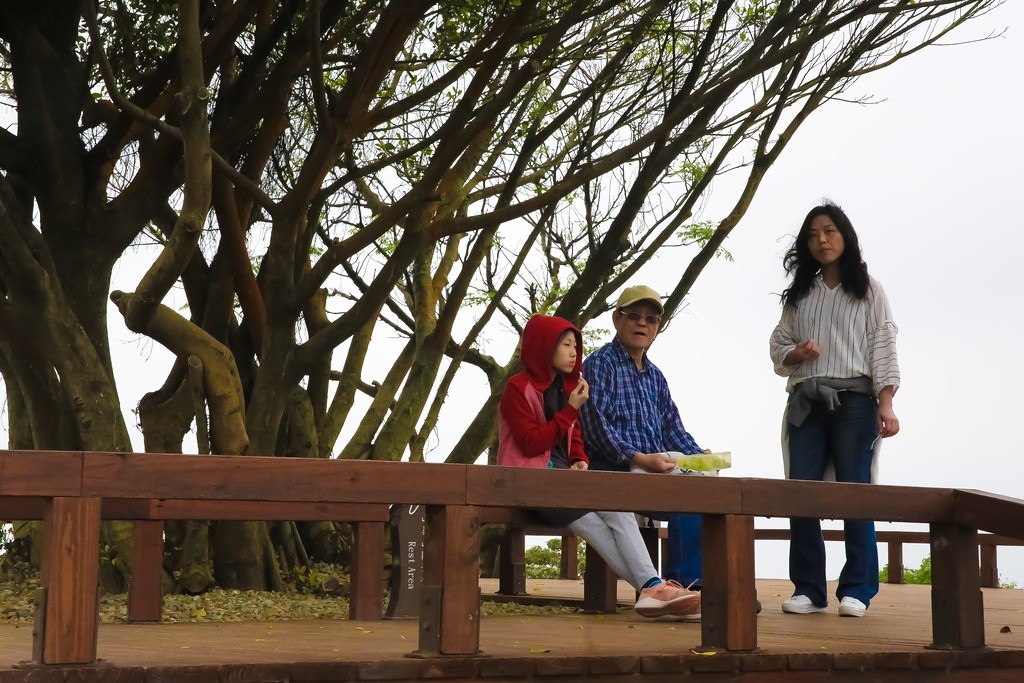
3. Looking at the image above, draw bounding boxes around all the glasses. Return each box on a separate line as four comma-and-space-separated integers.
619, 308, 662, 325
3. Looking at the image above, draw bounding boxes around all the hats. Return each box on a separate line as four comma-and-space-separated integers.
616, 284, 665, 313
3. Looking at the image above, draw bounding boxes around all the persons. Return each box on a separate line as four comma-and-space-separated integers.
498, 312, 707, 613
768, 203, 900, 616
582, 285, 765, 614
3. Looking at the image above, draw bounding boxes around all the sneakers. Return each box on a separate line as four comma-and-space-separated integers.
634, 578, 701, 623
782, 593, 829, 613
838, 596, 869, 616
